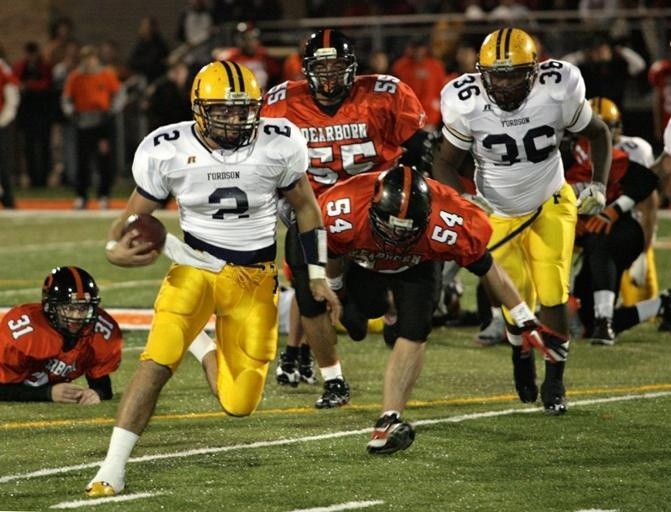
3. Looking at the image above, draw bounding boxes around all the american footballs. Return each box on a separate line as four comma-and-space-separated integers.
121, 214, 166, 256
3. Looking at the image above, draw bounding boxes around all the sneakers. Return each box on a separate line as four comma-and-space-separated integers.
366, 414, 416, 455
85, 473, 128, 496
301, 345, 316, 384
514, 376, 539, 402
655, 290, 671, 332
274, 346, 301, 386
315, 377, 351, 409
540, 382, 567, 415
474, 315, 505, 343
591, 315, 617, 348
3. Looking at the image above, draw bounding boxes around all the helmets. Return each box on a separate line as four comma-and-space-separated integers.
369, 166, 430, 255
477, 29, 537, 117
587, 98, 621, 142
191, 59, 260, 166
41, 266, 100, 338
303, 28, 357, 102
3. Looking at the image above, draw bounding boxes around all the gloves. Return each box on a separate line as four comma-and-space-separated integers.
574, 183, 607, 217
584, 195, 633, 234
510, 304, 571, 364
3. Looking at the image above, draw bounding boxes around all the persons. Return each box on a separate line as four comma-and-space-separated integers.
335, 270, 468, 333
98, 42, 128, 80
648, 119, 671, 333
432, 27, 613, 414
129, 16, 166, 80
0, 56, 21, 210
260, 28, 436, 387
84, 60, 343, 499
11, 41, 53, 189
562, 37, 646, 100
53, 38, 83, 186
559, 130, 658, 347
1, 265, 124, 406
42, 18, 79, 65
286, 167, 571, 456
221, 19, 276, 91
366, 36, 480, 127
62, 46, 119, 209
470, 270, 511, 346
588, 97, 658, 254
